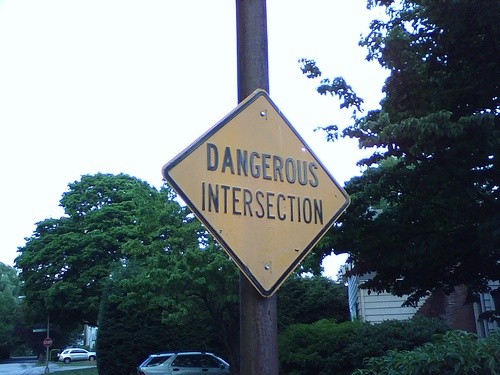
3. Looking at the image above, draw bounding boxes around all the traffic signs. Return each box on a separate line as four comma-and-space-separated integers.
33, 328, 48, 333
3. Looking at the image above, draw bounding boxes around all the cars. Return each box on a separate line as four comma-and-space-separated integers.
136, 351, 230, 375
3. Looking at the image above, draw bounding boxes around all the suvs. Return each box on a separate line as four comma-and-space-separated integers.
57, 348, 96, 364
